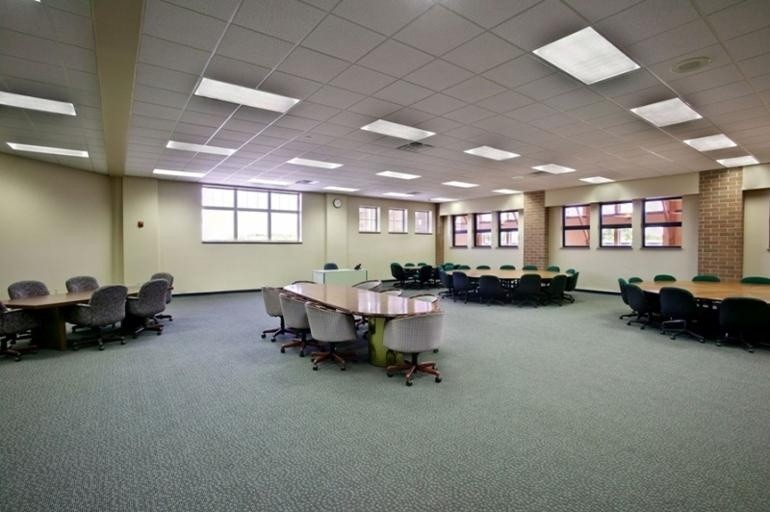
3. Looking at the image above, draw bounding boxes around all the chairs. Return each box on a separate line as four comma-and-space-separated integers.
1, 272, 174, 361
618, 274, 770, 353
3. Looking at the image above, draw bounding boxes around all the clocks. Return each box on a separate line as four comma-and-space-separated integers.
333, 198, 343, 208
